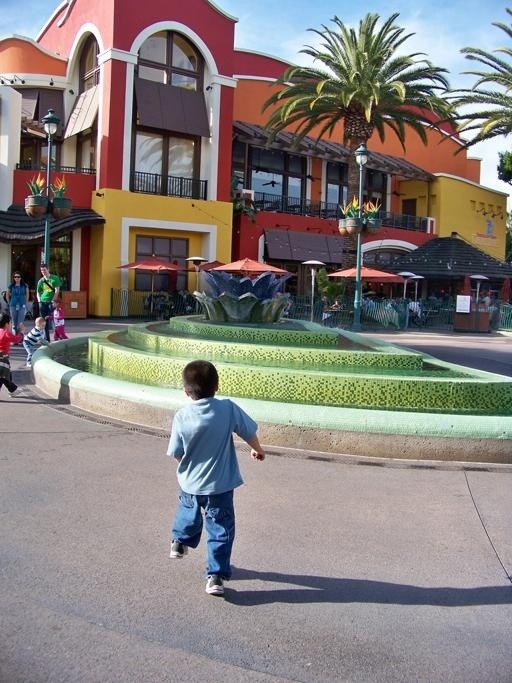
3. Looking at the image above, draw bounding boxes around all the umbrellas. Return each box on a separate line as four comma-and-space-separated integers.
188, 259, 229, 274
114, 253, 187, 316
209, 256, 289, 282
325, 264, 404, 305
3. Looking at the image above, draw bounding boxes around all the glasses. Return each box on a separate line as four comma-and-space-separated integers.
13, 275, 21, 279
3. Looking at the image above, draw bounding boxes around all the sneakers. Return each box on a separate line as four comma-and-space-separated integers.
8, 388, 23, 398
168, 540, 188, 559
204, 574, 224, 596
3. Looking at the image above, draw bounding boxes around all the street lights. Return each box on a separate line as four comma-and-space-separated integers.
469, 275, 488, 303
301, 260, 326, 322
398, 272, 424, 301
352, 144, 367, 332
41, 109, 60, 330
185, 256, 208, 314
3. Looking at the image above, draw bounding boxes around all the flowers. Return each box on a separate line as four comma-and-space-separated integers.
341, 195, 382, 218
25, 172, 70, 197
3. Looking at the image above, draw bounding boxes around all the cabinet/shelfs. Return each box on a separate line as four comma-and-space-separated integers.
58, 289, 88, 317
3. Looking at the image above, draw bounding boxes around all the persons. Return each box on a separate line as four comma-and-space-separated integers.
4, 269, 30, 338
1, 312, 29, 397
36, 263, 63, 341
164, 357, 266, 598
45, 296, 69, 340
23, 315, 53, 368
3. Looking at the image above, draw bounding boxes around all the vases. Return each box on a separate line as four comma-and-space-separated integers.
24, 196, 72, 222
338, 217, 382, 236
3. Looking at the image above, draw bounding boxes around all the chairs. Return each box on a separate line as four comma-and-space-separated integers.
292, 295, 451, 326
490, 301, 511, 325
